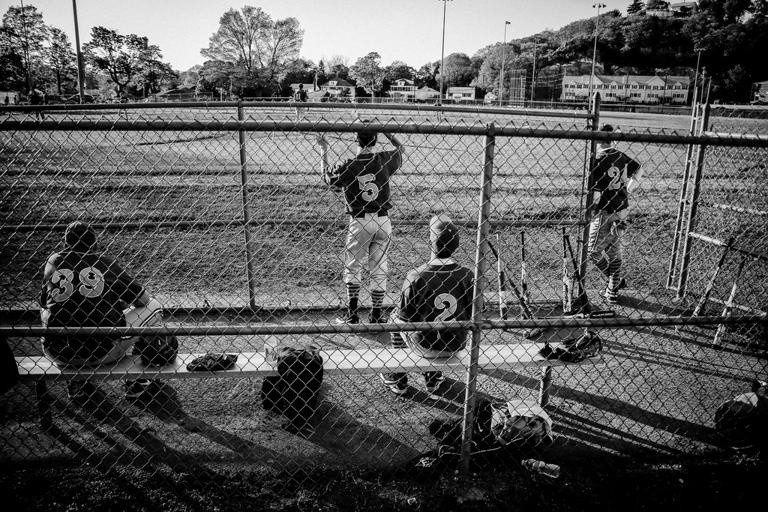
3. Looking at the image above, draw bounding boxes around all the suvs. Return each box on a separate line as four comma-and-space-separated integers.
19, 94, 67, 106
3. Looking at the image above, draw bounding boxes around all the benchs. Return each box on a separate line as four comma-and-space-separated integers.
16, 341, 602, 436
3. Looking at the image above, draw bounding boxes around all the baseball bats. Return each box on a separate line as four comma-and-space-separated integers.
487, 226, 615, 339
692, 236, 736, 316
712, 256, 747, 348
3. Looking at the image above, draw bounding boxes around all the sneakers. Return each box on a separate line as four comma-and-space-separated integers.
425, 371, 445, 391
380, 372, 407, 394
337, 312, 359, 324
600, 287, 618, 303
126, 378, 160, 397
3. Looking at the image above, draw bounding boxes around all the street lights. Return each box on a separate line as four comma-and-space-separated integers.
701, 66, 707, 103
692, 47, 707, 104
588, 4, 607, 112
499, 20, 512, 107
530, 32, 542, 107
438, 0, 453, 106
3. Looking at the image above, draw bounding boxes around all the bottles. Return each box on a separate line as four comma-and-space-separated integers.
264, 323, 278, 364
522, 459, 559, 478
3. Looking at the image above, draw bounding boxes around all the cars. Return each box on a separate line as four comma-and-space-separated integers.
67, 94, 94, 104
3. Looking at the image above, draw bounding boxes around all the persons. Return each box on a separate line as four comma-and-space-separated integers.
23, 88, 46, 122
378, 213, 475, 395
584, 124, 642, 305
32, 220, 163, 373
315, 119, 404, 325
292, 83, 308, 121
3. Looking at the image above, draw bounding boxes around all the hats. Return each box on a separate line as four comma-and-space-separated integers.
430, 215, 459, 248
